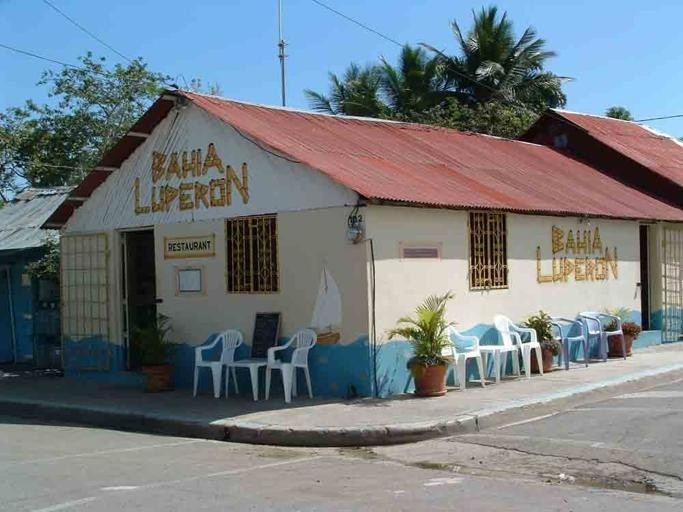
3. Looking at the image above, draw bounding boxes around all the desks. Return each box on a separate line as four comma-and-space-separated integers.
226, 358, 281, 401
464, 341, 519, 383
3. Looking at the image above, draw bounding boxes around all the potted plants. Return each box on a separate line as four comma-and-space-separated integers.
126, 314, 179, 395
602, 307, 642, 357
519, 312, 561, 375
387, 292, 459, 398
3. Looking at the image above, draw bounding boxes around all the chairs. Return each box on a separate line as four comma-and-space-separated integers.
194, 331, 243, 398
494, 314, 545, 378
579, 312, 628, 362
265, 329, 316, 403
439, 321, 486, 390
550, 318, 589, 371
